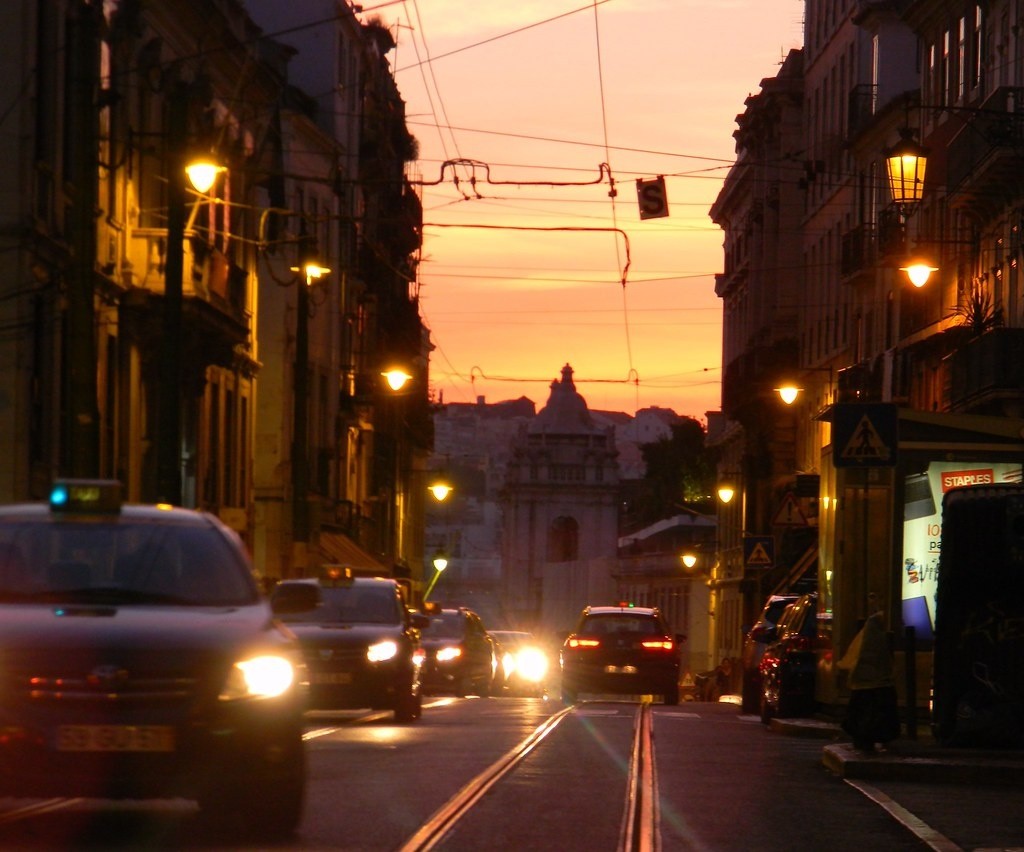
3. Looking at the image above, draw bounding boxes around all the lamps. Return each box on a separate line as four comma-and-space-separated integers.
883, 125, 928, 219
719, 471, 742, 503
680, 540, 720, 567
899, 229, 980, 289
771, 366, 833, 405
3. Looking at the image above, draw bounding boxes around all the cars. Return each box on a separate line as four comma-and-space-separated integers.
412, 606, 488, 697
273, 576, 428, 723
491, 631, 558, 698
564, 603, 682, 708
0, 475, 307, 852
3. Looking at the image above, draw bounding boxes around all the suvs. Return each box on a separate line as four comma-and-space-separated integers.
738, 595, 813, 710
760, 596, 855, 713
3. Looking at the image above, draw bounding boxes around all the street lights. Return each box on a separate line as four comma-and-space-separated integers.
158, 74, 230, 508
